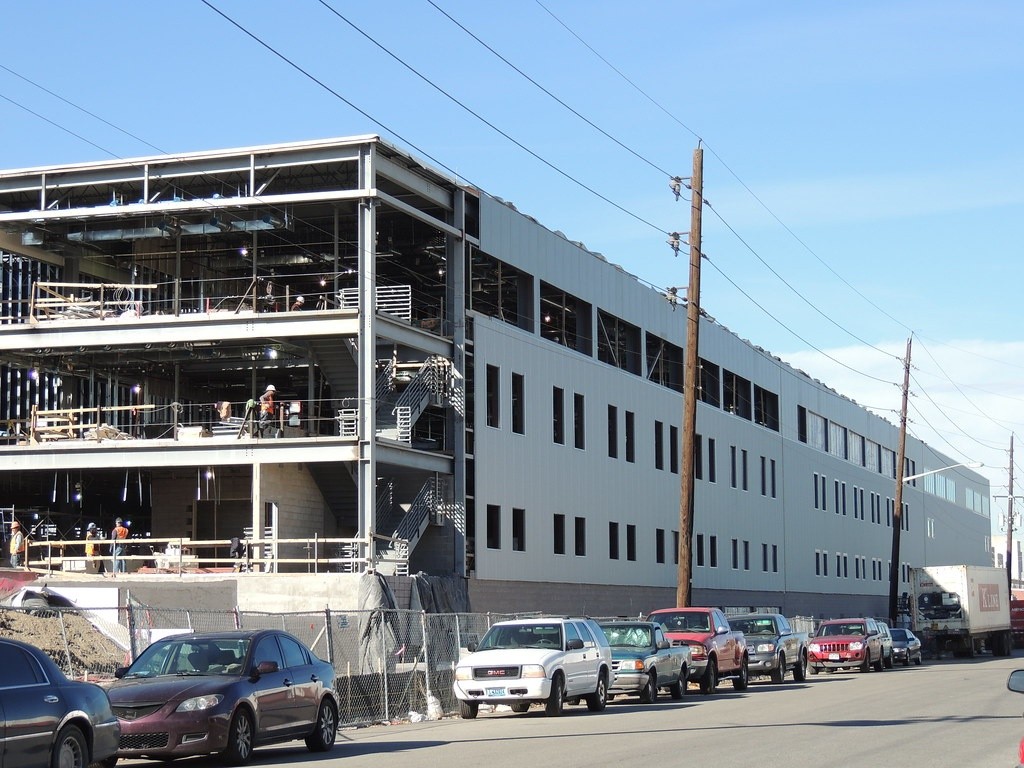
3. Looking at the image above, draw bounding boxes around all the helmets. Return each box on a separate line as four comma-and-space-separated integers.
10, 521, 20, 528
265, 384, 276, 391
296, 296, 305, 303
116, 517, 123, 522
87, 522, 96, 530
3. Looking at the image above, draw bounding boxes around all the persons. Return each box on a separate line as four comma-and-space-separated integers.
290, 296, 305, 311
86, 523, 107, 574
252, 384, 276, 438
9, 521, 26, 567
109, 517, 129, 574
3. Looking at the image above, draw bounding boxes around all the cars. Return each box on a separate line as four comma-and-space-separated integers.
807, 617, 921, 675
0, 629, 340, 768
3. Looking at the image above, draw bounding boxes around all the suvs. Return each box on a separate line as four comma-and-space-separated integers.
453, 615, 615, 719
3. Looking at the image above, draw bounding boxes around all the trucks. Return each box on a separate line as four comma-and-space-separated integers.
909, 565, 1012, 658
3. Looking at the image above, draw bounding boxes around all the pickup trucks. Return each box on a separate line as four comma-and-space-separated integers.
567, 608, 808, 706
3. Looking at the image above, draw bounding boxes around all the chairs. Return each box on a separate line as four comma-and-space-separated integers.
187, 652, 209, 671
623, 627, 649, 645
217, 649, 236, 665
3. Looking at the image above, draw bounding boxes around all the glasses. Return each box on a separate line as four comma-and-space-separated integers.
273, 390, 275, 392
